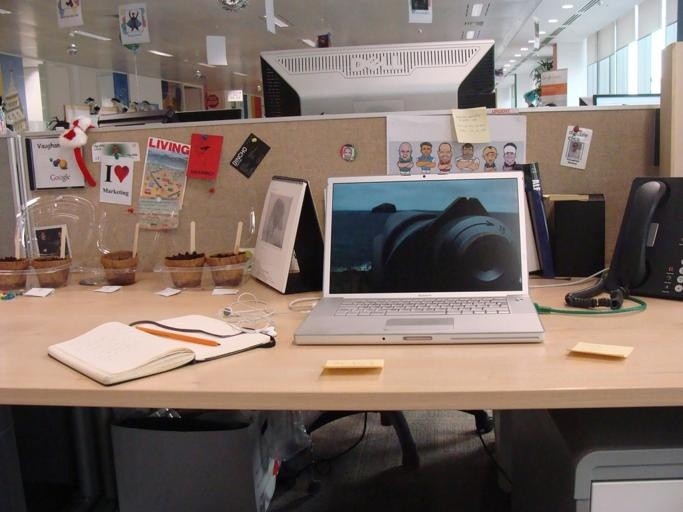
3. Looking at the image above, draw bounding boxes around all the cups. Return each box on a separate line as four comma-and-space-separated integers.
100, 252, 140, 285
31, 255, 72, 287
0, 256, 29, 291
165, 249, 205, 287
207, 250, 249, 289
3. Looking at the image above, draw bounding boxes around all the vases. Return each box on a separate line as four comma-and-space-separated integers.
0, 246, 247, 298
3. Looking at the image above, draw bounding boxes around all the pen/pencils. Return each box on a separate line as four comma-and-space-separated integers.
136, 326, 221, 346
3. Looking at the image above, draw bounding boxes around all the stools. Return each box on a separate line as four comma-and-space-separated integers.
299, 405, 493, 476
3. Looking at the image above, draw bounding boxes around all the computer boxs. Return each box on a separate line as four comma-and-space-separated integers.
491, 406, 683, 510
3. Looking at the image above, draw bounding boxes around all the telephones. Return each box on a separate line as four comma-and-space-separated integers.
604, 177, 683, 301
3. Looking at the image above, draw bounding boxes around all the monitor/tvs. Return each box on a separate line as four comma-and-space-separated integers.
167, 108, 242, 123
593, 94, 662, 106
257, 37, 496, 119
98, 108, 166, 126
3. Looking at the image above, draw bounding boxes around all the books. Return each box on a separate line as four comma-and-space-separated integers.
47, 313, 271, 387
512, 156, 606, 280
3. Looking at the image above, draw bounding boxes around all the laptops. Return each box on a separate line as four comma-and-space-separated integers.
293, 170, 546, 347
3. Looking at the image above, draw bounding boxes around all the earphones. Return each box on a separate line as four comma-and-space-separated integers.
255, 324, 278, 338
221, 305, 233, 317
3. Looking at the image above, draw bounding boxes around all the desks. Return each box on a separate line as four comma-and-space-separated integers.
0, 99, 682, 511
